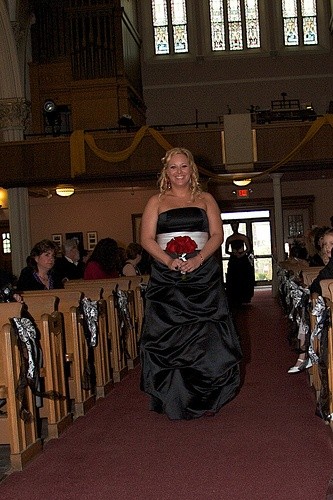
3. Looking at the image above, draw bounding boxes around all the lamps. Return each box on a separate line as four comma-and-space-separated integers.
43, 100, 62, 137
232, 179, 252, 187
55, 189, 75, 197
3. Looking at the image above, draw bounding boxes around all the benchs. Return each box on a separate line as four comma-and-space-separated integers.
0, 260, 333, 471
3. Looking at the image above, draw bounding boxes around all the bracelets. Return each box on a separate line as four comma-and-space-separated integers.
198, 252, 204, 265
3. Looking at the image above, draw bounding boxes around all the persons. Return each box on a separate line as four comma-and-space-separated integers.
224, 220, 254, 305
285, 216, 333, 374
136, 146, 246, 421
16, 239, 63, 293
59, 239, 152, 281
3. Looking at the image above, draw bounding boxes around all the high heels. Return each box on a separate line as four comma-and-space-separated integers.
287, 358, 304, 374
